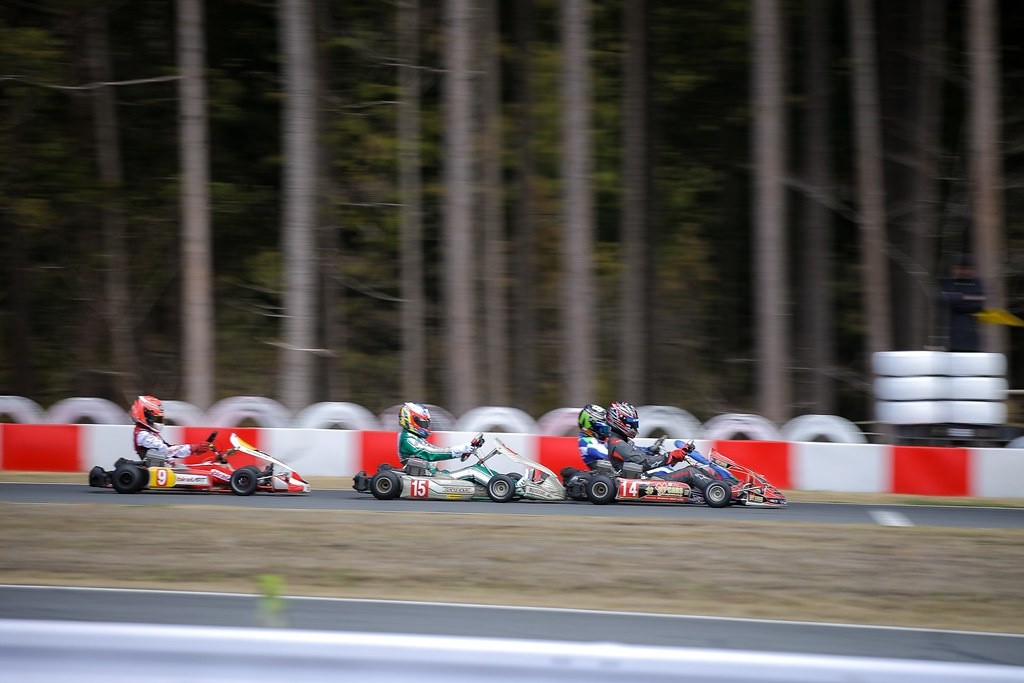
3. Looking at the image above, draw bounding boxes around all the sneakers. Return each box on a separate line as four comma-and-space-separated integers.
729, 480, 746, 497
528, 467, 536, 483
516, 468, 530, 487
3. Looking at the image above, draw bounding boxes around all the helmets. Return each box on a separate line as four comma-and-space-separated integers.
398, 401, 432, 439
606, 401, 639, 439
129, 394, 165, 434
579, 403, 608, 441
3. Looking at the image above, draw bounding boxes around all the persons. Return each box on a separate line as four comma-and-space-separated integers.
130, 396, 274, 486
397, 402, 535, 498
578, 404, 742, 481
603, 401, 753, 502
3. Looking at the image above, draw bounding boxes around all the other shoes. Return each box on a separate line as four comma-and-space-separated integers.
254, 466, 272, 477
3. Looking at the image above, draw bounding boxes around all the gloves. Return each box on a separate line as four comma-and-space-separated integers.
665, 449, 687, 465
189, 441, 212, 453
451, 443, 477, 457
